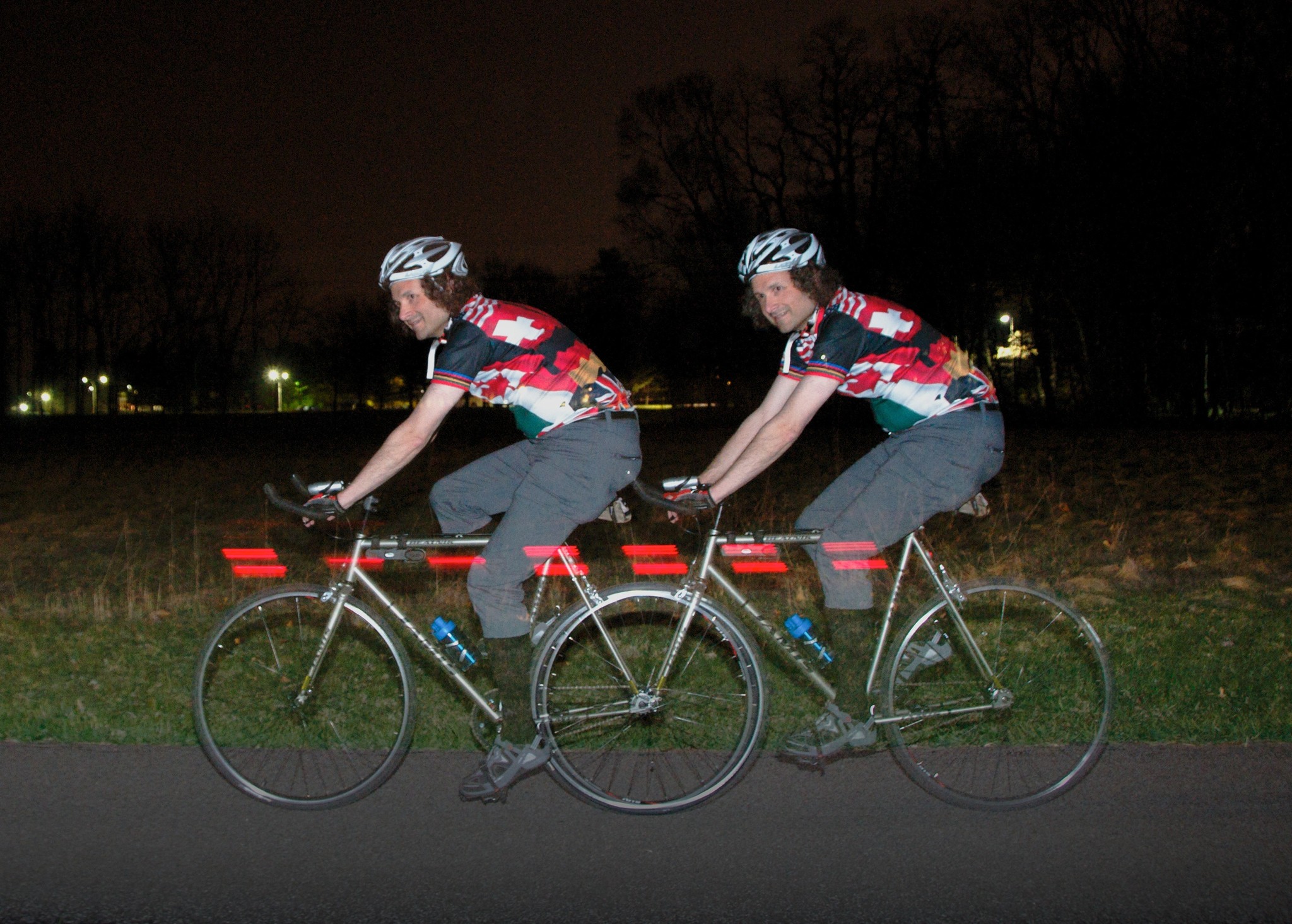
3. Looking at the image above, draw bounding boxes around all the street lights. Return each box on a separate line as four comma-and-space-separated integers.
268, 369, 289, 413
82, 376, 98, 415
999, 313, 1014, 401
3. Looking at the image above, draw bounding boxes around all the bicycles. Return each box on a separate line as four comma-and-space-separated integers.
187, 462, 769, 818
536, 475, 1115, 813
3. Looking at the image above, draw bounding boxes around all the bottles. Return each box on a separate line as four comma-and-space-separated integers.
430, 616, 482, 672
784, 614, 835, 668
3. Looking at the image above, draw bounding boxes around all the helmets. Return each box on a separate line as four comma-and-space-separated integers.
737, 227, 826, 282
378, 237, 469, 291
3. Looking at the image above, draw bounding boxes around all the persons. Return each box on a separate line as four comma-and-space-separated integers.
300, 235, 641, 803
648, 225, 1005, 761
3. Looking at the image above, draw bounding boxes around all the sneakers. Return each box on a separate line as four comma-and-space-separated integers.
784, 701, 877, 758
876, 631, 952, 700
459, 732, 552, 797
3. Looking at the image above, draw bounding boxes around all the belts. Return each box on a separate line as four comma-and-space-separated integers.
572, 411, 637, 423
965, 402, 1000, 413
